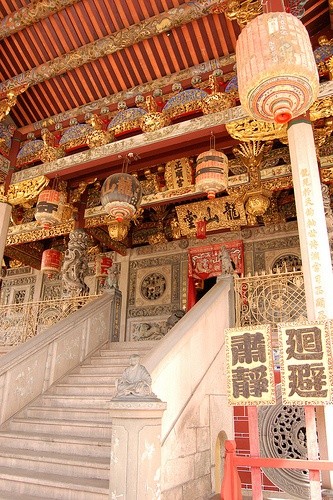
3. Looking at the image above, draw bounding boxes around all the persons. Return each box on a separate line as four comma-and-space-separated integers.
218, 244, 238, 274
105, 262, 120, 290
